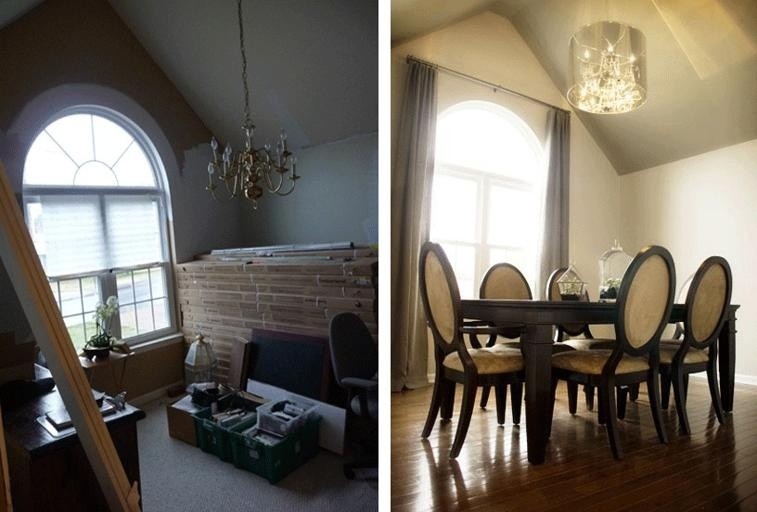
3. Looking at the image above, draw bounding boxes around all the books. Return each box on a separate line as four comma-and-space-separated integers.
45, 397, 116, 430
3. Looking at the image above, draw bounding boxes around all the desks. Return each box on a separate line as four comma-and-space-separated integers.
458, 299, 740, 465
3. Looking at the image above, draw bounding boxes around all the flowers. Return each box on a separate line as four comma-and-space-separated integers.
84, 295, 119, 346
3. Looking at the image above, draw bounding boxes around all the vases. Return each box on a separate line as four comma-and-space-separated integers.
83, 348, 110, 362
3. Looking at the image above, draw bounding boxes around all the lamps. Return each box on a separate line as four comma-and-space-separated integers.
203, 1, 300, 210
564, 21, 648, 115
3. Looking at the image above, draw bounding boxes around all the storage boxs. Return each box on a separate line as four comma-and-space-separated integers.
165, 390, 323, 485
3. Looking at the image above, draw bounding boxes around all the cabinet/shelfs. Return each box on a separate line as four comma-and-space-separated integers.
0, 362, 146, 512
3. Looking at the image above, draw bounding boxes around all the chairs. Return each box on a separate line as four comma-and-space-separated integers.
327, 310, 377, 480
